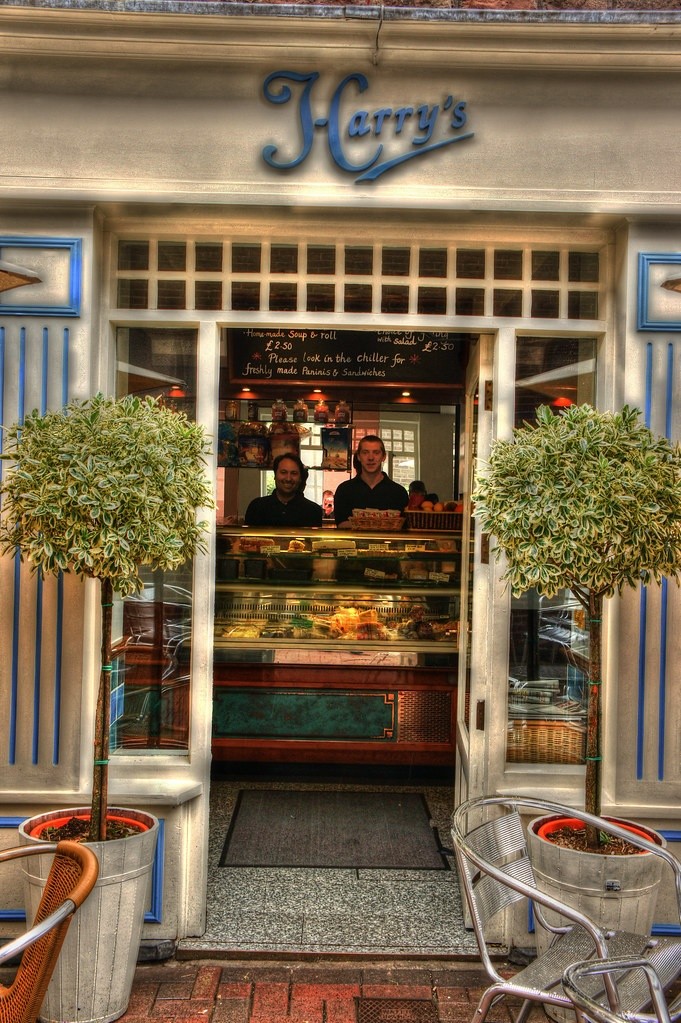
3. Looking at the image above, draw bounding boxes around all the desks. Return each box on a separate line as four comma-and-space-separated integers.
510, 586, 590, 702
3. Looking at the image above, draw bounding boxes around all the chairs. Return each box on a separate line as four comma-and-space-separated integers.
521, 587, 590, 677
507, 719, 587, 764
107, 642, 179, 735
447, 795, 681, 1023
108, 675, 191, 751
0, 839, 99, 1023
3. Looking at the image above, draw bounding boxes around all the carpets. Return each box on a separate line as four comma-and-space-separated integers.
218, 788, 452, 873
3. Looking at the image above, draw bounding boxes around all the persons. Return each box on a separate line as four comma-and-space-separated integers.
318, 490, 335, 521
334, 436, 410, 584
244, 453, 324, 588
406, 480, 441, 509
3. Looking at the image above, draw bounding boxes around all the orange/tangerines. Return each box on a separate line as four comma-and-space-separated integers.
421, 501, 444, 512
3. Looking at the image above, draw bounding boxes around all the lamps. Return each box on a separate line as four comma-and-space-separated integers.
391, 389, 419, 404
301, 387, 335, 402
168, 385, 186, 397
231, 386, 262, 399
552, 397, 573, 406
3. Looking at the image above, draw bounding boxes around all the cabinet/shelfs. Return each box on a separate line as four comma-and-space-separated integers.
217, 527, 464, 770
218, 398, 354, 474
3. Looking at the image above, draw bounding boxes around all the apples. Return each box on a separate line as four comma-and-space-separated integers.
455, 504, 464, 513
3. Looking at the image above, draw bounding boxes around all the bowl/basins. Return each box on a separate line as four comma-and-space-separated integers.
242, 559, 267, 579
312, 559, 338, 581
268, 568, 312, 581
216, 558, 240, 580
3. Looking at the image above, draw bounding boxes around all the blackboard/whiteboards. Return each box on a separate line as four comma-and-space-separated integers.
228, 295, 473, 388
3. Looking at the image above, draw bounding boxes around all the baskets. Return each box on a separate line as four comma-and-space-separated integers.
348, 517, 406, 532
506, 720, 587, 765
404, 510, 463, 531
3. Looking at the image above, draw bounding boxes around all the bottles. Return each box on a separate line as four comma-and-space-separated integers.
271, 397, 287, 421
294, 398, 308, 421
314, 398, 330, 422
248, 400, 258, 419
225, 400, 237, 419
334, 400, 350, 423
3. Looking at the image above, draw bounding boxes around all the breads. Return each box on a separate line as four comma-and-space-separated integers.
239, 537, 305, 553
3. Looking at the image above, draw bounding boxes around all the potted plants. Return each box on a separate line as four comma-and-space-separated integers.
0, 391, 220, 1023
467, 403, 681, 1023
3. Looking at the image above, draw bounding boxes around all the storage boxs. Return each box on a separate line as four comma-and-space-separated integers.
407, 569, 428, 581
400, 560, 439, 579
437, 540, 458, 551
440, 561, 458, 573
404, 504, 474, 533
216, 558, 239, 581
237, 436, 270, 468
321, 427, 350, 469
270, 434, 299, 468
242, 557, 268, 581
